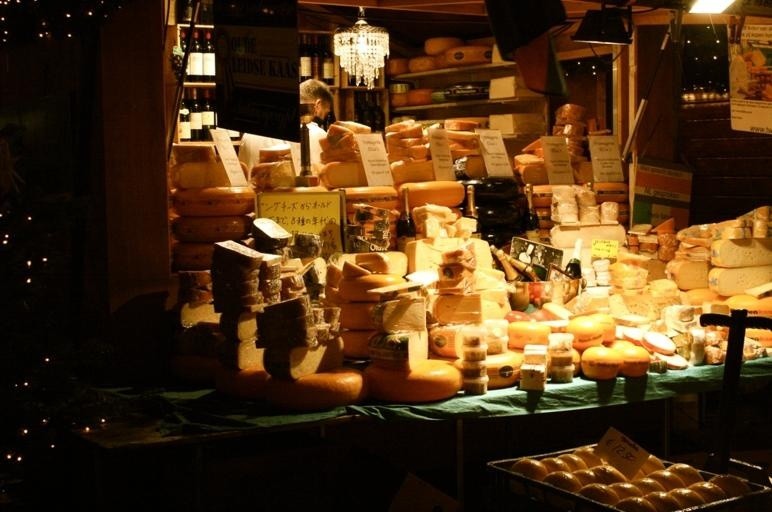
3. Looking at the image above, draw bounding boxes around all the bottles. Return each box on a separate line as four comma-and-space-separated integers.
565, 239, 584, 280
203, 33, 216, 82
508, 255, 546, 282
313, 34, 321, 81
464, 184, 485, 240
180, 32, 191, 79
321, 36, 334, 86
190, 31, 203, 82
495, 249, 530, 281
522, 182, 540, 234
203, 88, 218, 141
179, 88, 191, 141
300, 34, 313, 83
354, 91, 385, 132
191, 88, 203, 141
396, 188, 417, 251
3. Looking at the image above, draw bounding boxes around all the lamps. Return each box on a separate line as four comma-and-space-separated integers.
572, 7, 633, 45
332, 5, 391, 90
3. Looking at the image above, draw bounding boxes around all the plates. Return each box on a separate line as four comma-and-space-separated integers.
486, 443, 772, 512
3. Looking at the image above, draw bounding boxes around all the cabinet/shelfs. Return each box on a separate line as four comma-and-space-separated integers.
173, 22, 389, 147
390, 58, 548, 140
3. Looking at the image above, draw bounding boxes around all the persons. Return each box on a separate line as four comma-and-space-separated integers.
299, 79, 335, 178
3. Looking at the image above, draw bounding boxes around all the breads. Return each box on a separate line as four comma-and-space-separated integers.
709, 235, 772, 268
171, 160, 249, 190
664, 259, 712, 292
250, 160, 296, 188
429, 319, 509, 358
173, 187, 256, 217
237, 336, 266, 370
404, 238, 493, 274
390, 159, 435, 183
366, 330, 429, 371
518, 163, 548, 185
318, 160, 368, 189
178, 302, 223, 328
372, 296, 427, 334
708, 264, 772, 296
172, 240, 247, 270
271, 337, 345, 381
219, 313, 258, 341
170, 215, 253, 243
338, 250, 409, 277
433, 290, 512, 326
